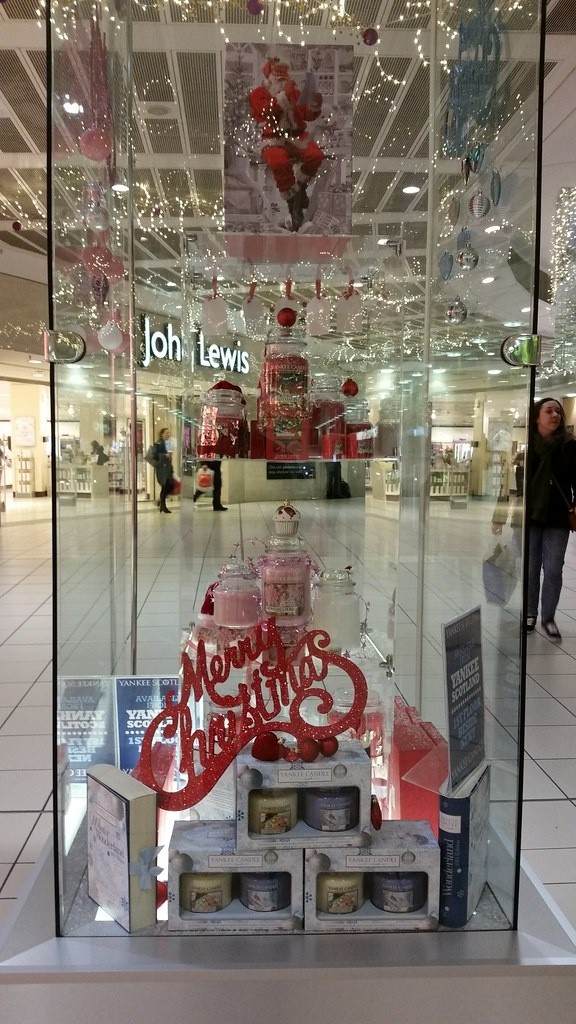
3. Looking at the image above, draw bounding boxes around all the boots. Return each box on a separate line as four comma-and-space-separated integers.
160, 504, 171, 513
157, 501, 160, 506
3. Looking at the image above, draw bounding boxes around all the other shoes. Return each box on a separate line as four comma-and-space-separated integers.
193, 491, 199, 502
214, 506, 228, 511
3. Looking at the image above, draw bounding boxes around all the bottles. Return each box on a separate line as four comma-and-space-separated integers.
430, 484, 444, 493
214, 560, 260, 627
314, 569, 359, 647
365, 467, 370, 483
260, 537, 311, 627
430, 473, 443, 484
449, 483, 465, 494
445, 473, 466, 483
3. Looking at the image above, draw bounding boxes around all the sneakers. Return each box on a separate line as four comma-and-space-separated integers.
541, 622, 562, 642
527, 616, 537, 633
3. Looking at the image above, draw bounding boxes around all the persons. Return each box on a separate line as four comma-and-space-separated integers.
192, 460, 228, 511
324, 461, 341, 498
153, 427, 174, 513
249, 53, 324, 233
90, 440, 109, 465
491, 397, 576, 644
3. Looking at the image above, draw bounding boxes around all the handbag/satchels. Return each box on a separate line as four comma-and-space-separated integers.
196, 468, 214, 492
483, 534, 518, 605
144, 441, 164, 468
568, 502, 576, 533
98, 448, 109, 465
